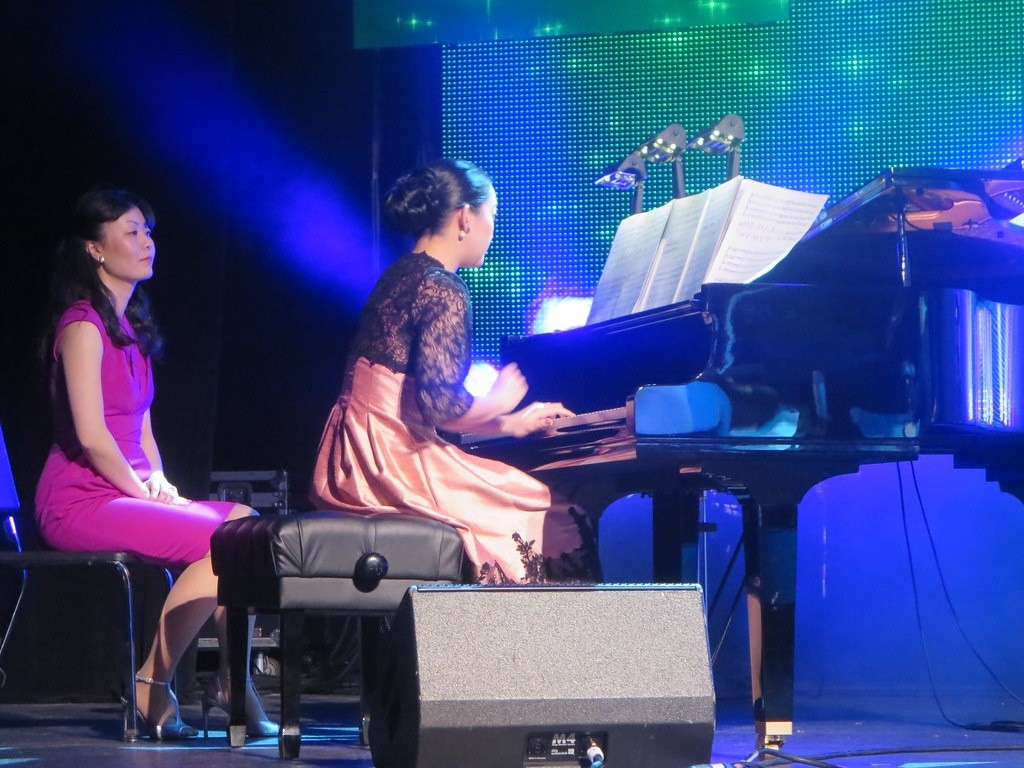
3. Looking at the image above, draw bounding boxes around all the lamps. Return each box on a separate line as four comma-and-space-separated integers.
686, 115, 744, 181
634, 124, 687, 200
596, 153, 646, 216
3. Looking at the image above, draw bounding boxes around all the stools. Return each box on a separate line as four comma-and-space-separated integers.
211, 510, 463, 758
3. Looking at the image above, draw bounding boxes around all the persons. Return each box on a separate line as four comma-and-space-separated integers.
34, 198, 280, 739
310, 159, 598, 580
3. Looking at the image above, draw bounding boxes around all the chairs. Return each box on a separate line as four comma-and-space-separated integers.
0, 425, 177, 742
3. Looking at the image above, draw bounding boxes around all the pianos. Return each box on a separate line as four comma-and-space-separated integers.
458, 166, 1024, 736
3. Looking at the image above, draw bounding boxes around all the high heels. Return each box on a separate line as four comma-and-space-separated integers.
201, 672, 278, 737
118, 671, 200, 738
